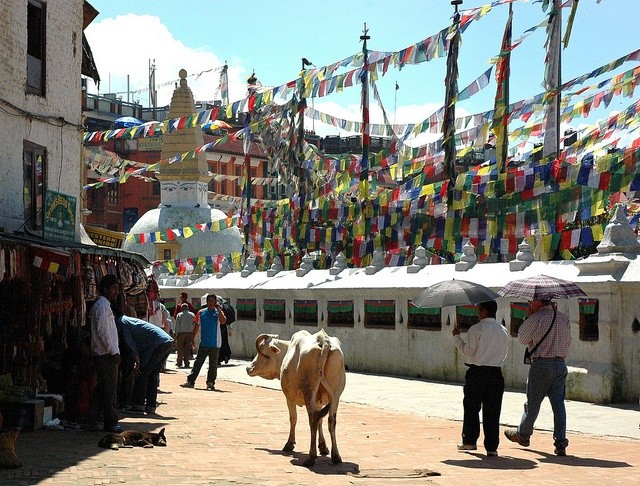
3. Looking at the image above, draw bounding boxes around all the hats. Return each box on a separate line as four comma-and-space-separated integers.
98, 274, 120, 286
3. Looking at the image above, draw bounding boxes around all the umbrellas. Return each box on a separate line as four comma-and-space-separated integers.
219, 303, 237, 323
200, 120, 231, 130
408, 280, 502, 326
498, 273, 588, 302
113, 115, 143, 126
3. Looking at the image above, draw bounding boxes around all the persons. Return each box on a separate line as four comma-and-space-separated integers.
175, 303, 196, 367
111, 305, 174, 415
218, 324, 232, 365
180, 295, 227, 390
451, 300, 511, 457
81, 273, 125, 433
160, 298, 172, 335
148, 282, 168, 372
503, 296, 573, 458
174, 291, 196, 360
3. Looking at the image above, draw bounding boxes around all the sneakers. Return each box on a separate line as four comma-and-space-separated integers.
504, 429, 531, 447
180, 381, 194, 388
554, 446, 564, 456
103, 425, 125, 434
457, 443, 477, 451
206, 383, 215, 391
78, 424, 104, 432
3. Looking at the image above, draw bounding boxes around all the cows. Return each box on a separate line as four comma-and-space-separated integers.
246, 328, 346, 466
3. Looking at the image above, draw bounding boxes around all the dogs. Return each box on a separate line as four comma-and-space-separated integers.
99, 426, 168, 451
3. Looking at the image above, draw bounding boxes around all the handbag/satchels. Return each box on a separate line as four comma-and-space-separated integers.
523, 347, 532, 365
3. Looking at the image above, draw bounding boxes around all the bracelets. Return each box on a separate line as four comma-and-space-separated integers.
134, 360, 140, 365
217, 309, 223, 312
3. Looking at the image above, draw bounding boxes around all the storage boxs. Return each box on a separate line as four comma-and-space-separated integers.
16, 401, 44, 430
43, 406, 52, 425
